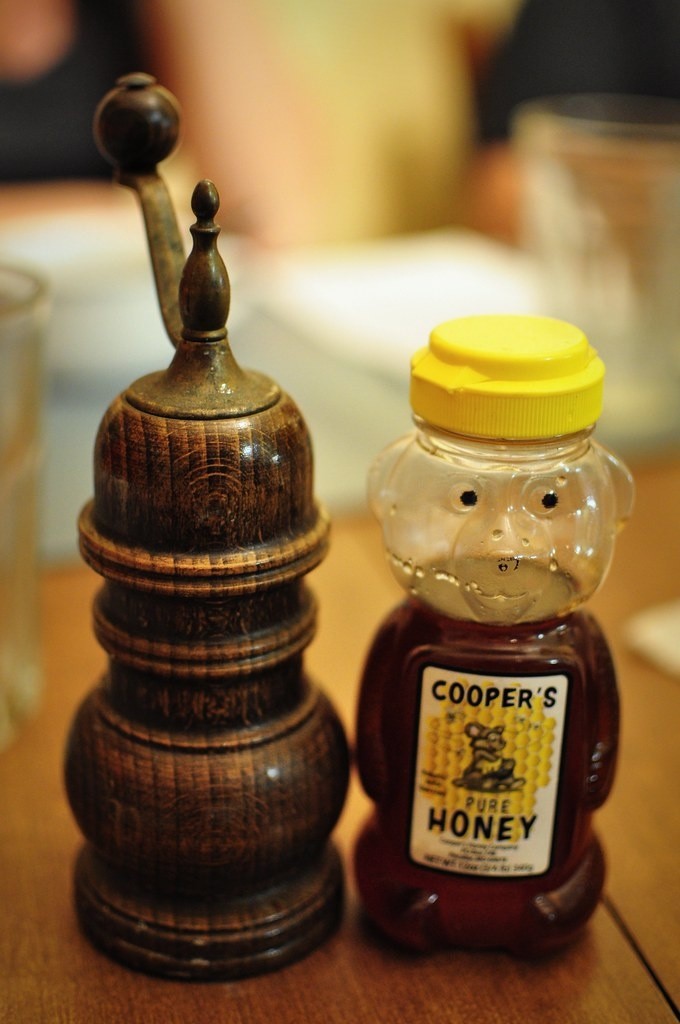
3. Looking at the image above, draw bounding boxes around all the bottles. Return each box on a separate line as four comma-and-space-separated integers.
347, 310, 636, 956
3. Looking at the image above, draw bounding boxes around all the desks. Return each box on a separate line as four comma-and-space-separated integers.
0, 446, 680, 1024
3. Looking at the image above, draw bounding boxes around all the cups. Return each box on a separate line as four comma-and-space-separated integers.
515, 89, 680, 469
0, 262, 48, 746
